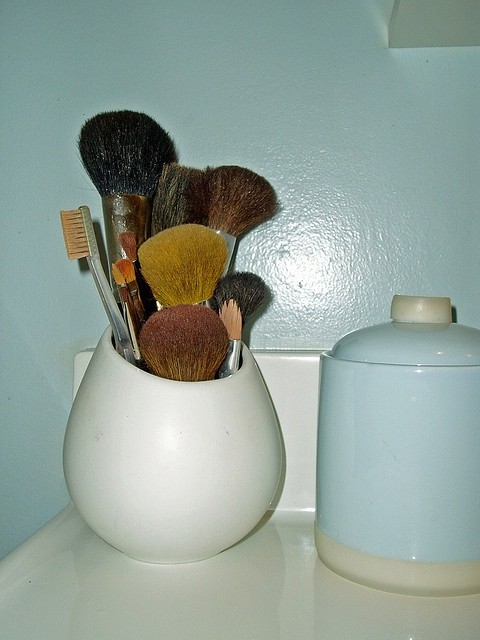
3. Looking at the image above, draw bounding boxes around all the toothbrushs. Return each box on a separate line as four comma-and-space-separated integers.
60, 205, 138, 367
